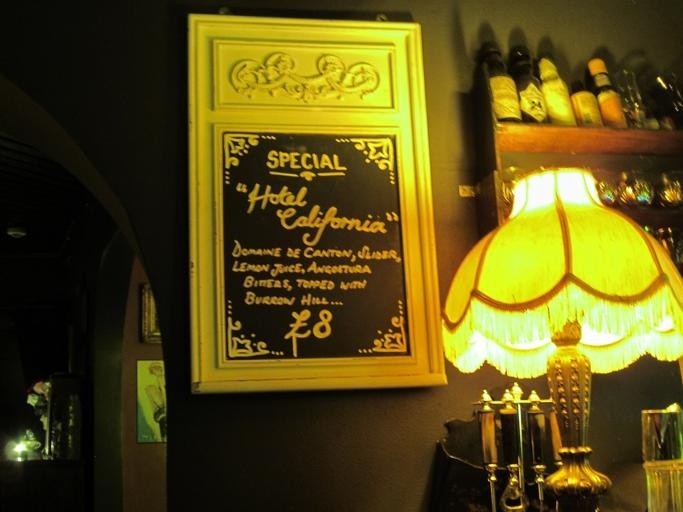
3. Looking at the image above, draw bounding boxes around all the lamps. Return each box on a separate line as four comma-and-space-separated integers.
428, 166, 681, 512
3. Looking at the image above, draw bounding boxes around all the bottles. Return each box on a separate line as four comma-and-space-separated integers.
507, 40, 548, 125
568, 78, 604, 129
655, 73, 683, 127
481, 38, 522, 125
536, 51, 577, 129
642, 221, 683, 263
584, 56, 629, 129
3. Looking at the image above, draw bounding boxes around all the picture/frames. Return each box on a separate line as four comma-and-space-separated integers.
142, 284, 162, 346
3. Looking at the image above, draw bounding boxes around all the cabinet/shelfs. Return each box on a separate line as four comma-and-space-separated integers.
470, 64, 683, 264
